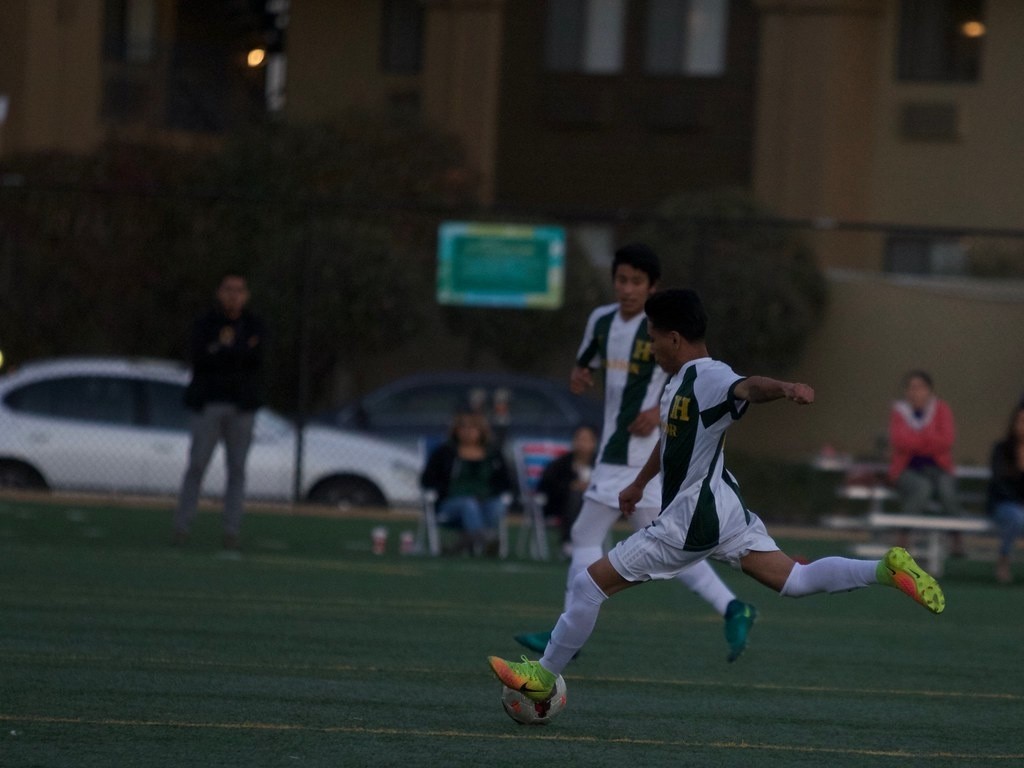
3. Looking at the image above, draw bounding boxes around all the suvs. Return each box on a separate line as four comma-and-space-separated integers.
0, 359, 440, 510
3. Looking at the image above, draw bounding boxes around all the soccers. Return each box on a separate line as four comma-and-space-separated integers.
500, 657, 566, 729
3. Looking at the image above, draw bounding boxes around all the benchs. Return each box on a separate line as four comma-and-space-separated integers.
814, 462, 997, 579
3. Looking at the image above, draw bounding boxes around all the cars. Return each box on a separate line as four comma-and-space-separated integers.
345, 370, 594, 444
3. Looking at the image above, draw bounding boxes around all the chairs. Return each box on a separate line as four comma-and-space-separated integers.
416, 436, 512, 560
512, 439, 570, 562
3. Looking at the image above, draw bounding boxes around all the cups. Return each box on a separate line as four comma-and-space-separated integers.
401, 534, 414, 555
372, 529, 387, 554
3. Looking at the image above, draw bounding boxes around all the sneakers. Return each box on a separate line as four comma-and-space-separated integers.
723, 598, 756, 663
883, 545, 946, 615
487, 653, 557, 702
511, 628, 582, 660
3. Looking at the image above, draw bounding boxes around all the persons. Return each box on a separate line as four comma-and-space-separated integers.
489, 288, 944, 701
987, 400, 1024, 587
888, 368, 968, 563
164, 265, 280, 537
418, 383, 602, 557
513, 241, 757, 659
